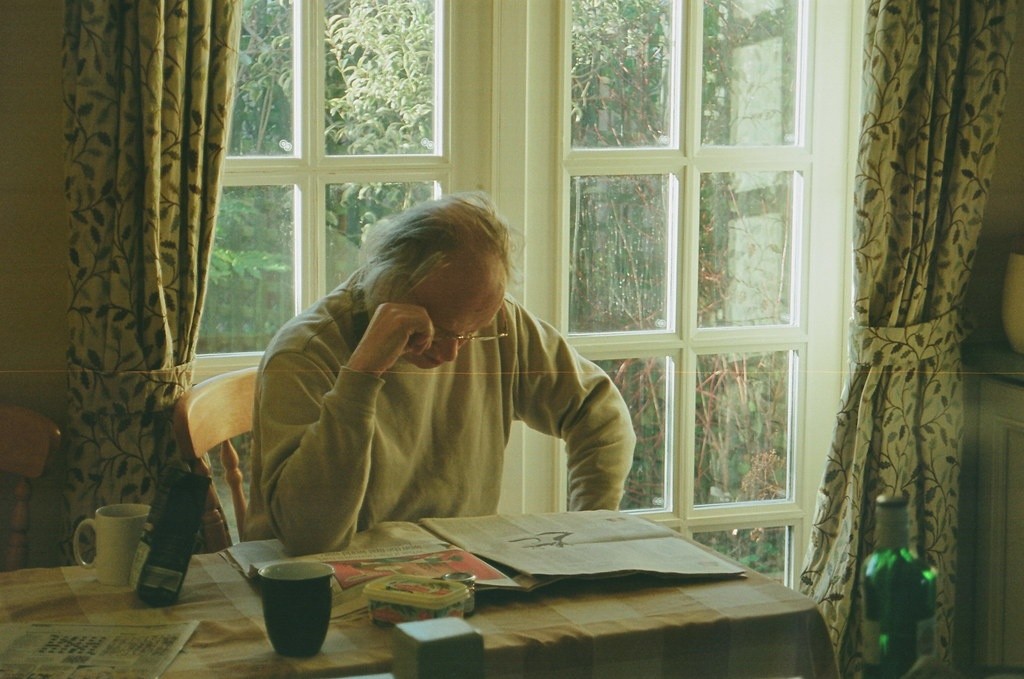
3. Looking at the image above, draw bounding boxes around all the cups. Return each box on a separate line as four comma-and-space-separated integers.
74, 504, 151, 586
256, 562, 336, 657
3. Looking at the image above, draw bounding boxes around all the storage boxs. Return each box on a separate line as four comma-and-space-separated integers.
361, 573, 468, 632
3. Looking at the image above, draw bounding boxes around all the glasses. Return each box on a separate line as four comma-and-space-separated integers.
405, 273, 509, 343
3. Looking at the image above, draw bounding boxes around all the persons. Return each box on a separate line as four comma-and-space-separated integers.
244, 196, 636, 558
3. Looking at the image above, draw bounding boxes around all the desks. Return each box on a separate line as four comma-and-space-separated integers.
0, 514, 839, 679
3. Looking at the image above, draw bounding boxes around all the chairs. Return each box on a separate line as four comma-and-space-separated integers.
0, 401, 62, 571
171, 367, 258, 552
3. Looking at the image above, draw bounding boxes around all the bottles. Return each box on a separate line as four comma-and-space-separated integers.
857, 495, 938, 679
